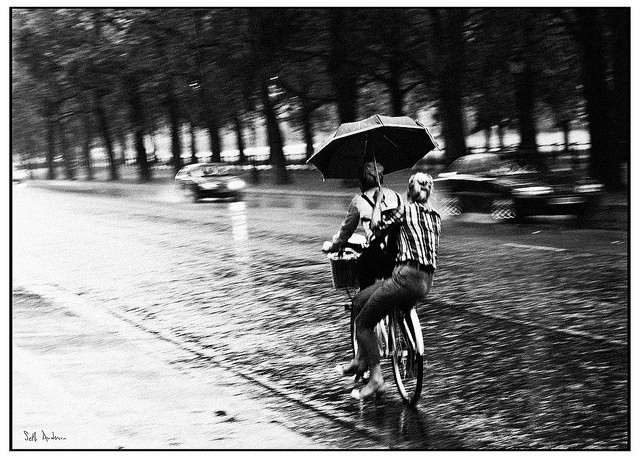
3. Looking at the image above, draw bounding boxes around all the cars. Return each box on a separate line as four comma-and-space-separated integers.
174, 162, 246, 202
11, 160, 31, 183
434, 152, 605, 222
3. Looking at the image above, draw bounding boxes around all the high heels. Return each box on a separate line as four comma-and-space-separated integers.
337, 359, 367, 382
350, 382, 385, 403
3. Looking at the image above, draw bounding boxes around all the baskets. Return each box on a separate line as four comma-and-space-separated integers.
328, 250, 362, 288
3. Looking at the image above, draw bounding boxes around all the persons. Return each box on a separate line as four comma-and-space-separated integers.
321, 161, 404, 292
342, 172, 441, 400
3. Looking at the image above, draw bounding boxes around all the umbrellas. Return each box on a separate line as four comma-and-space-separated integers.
306, 114, 435, 207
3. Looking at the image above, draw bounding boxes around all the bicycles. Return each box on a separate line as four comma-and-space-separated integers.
321, 234, 426, 407
